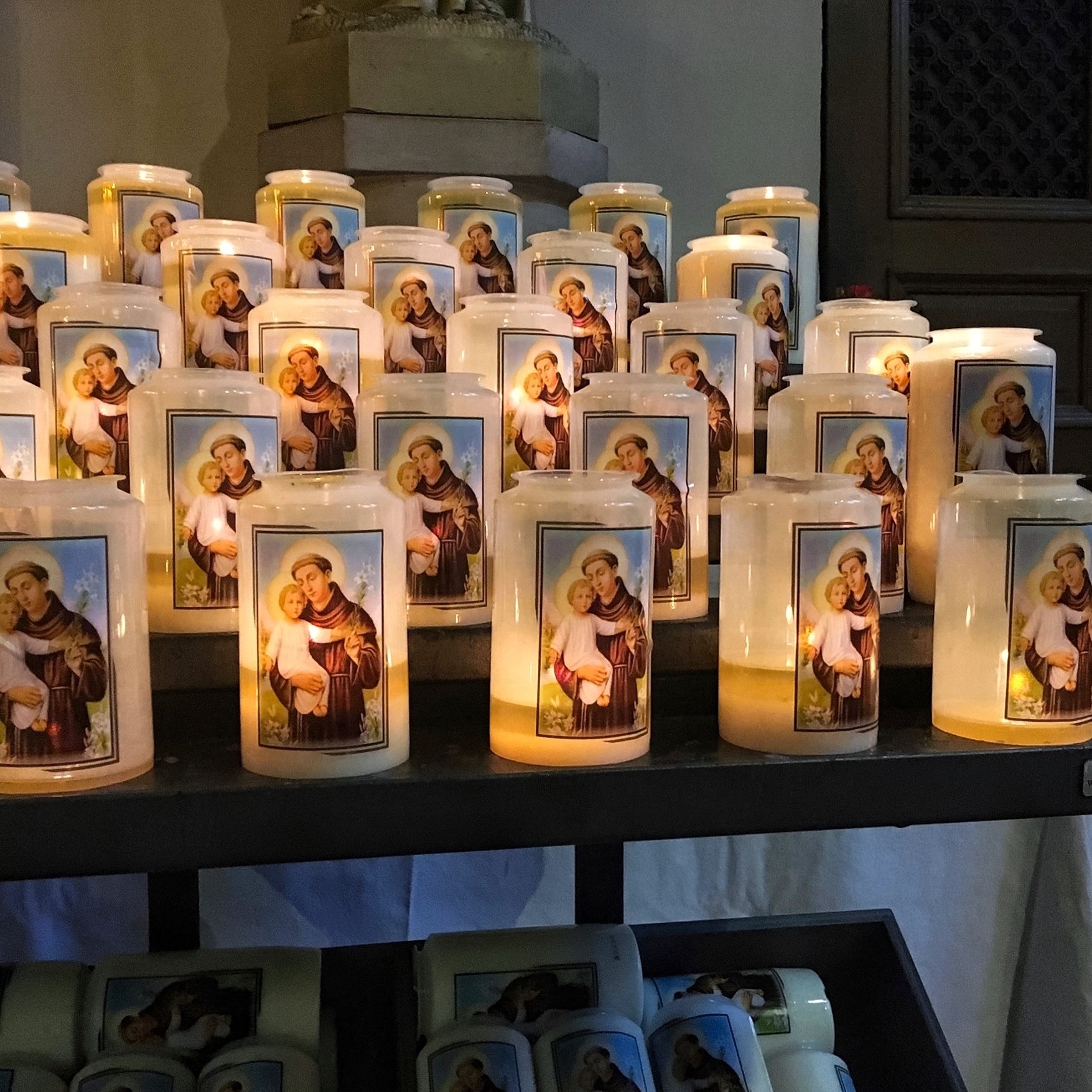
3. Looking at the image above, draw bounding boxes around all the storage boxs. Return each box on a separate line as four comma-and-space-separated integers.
321, 902, 976, 1091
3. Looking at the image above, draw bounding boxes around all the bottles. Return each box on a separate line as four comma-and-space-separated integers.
487, 468, 654, 764
0, 475, 152, 795
230, 470, 417, 779
929, 467, 1092, 745
717, 471, 886, 757
0, 157, 1063, 637
0, 924, 858, 1091
